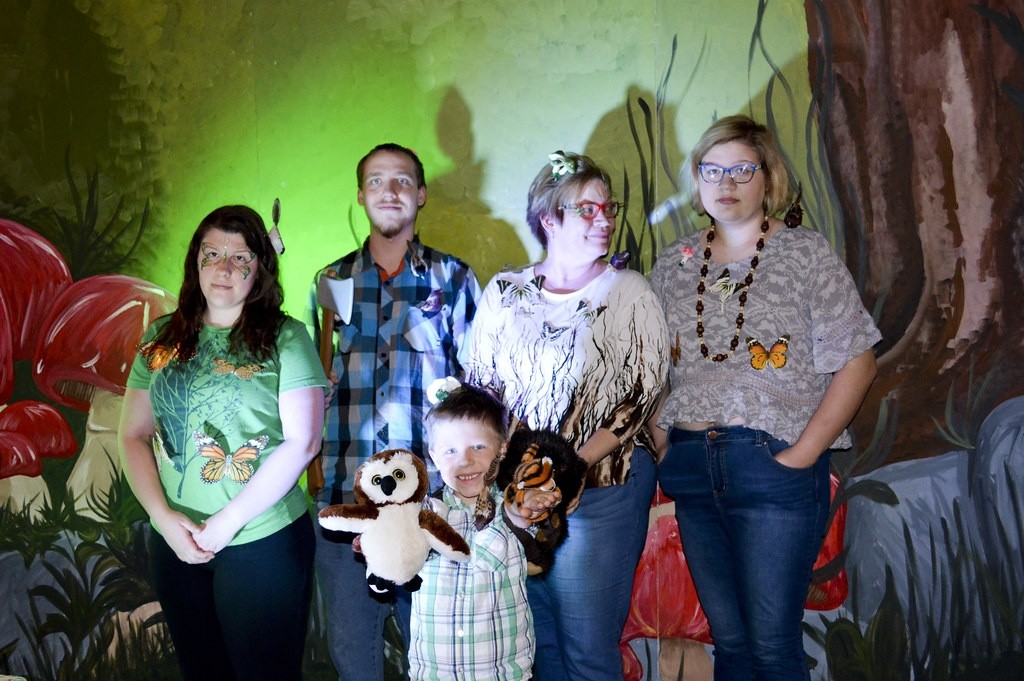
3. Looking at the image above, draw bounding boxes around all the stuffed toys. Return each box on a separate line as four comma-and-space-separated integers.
502, 443, 559, 523
494, 425, 589, 576
315, 450, 471, 600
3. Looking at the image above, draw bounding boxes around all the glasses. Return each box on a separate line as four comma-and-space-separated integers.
557, 199, 621, 219
697, 163, 765, 184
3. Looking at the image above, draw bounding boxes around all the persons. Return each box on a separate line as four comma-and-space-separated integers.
308, 144, 489, 681
455, 150, 671, 680
116, 203, 332, 680
643, 114, 885, 681
350, 382, 536, 681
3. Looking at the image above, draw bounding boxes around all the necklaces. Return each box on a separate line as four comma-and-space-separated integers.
695, 205, 769, 362
